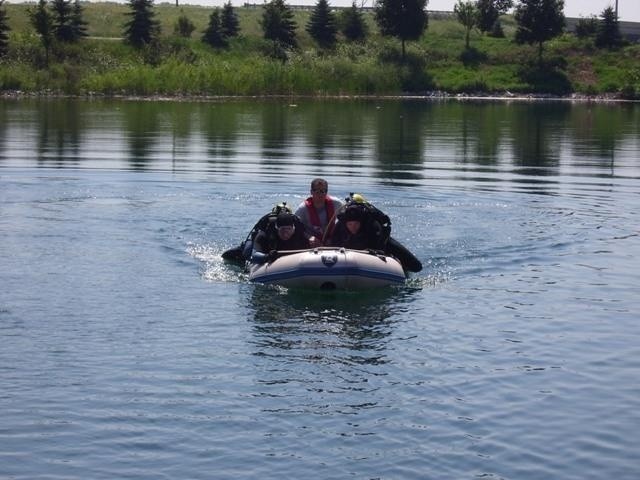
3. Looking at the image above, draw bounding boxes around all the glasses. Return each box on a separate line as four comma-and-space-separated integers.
273, 222, 297, 234
310, 188, 328, 195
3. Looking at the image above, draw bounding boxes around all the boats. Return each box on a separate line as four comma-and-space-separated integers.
245, 204, 405, 290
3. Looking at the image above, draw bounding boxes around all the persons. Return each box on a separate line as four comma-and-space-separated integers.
292, 178, 345, 250
328, 203, 422, 273
221, 211, 311, 266
